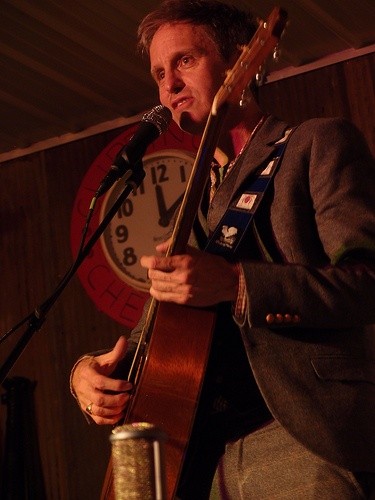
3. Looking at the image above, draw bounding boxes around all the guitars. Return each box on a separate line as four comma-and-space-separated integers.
102, 3, 288, 500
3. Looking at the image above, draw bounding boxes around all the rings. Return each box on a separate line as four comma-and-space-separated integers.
87, 403, 95, 414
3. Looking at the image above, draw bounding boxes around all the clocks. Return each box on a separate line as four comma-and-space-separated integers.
69, 119, 206, 330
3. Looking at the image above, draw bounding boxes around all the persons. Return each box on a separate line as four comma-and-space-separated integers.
68, 1, 375, 500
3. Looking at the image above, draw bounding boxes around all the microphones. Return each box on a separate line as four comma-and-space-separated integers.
95, 105, 172, 196
